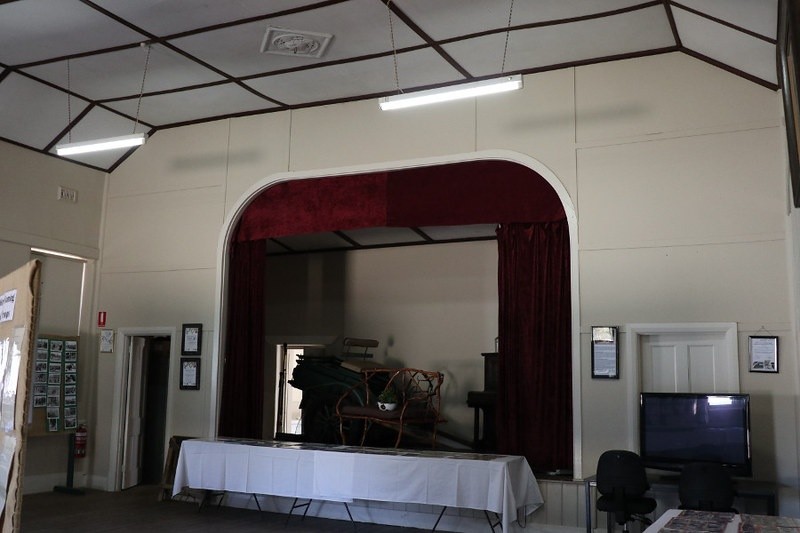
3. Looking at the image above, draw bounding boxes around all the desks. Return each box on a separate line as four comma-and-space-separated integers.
172, 435, 544, 533
643, 510, 800, 533
584, 472, 778, 533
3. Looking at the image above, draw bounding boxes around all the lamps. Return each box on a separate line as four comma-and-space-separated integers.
378, 0, 525, 111
55, 41, 151, 156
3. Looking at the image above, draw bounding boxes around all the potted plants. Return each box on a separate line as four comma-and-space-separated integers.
377, 387, 396, 412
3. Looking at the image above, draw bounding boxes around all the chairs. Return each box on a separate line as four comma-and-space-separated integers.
677, 462, 739, 513
340, 338, 392, 374
597, 450, 657, 533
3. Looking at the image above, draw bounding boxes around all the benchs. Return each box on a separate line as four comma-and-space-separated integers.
335, 367, 448, 450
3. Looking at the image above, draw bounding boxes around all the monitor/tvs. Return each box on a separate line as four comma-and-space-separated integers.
640, 392, 750, 484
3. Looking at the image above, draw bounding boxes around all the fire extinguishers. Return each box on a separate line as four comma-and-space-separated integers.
74, 423, 86, 459
